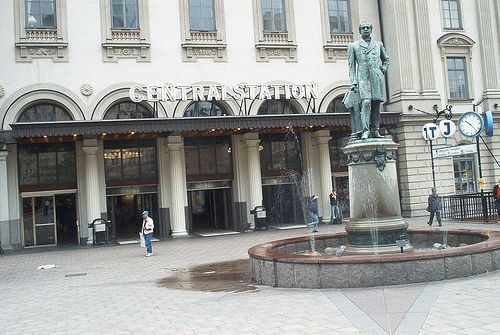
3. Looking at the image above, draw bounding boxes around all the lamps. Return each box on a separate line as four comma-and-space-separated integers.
28, 16, 37, 28
396, 239, 407, 248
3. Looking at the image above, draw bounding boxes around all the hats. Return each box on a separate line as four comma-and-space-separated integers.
142, 211, 148, 215
313, 196, 319, 199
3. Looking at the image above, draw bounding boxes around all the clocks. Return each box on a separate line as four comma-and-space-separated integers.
457, 111, 484, 138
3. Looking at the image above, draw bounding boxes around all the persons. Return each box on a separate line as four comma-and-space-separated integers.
329, 189, 340, 224
347, 21, 390, 138
56, 199, 76, 243
141, 210, 154, 257
43, 199, 50, 216
493, 176, 500, 224
426, 188, 443, 227
306, 195, 320, 232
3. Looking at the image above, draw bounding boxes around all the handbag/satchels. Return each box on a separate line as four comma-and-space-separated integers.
139, 232, 146, 247
426, 207, 432, 212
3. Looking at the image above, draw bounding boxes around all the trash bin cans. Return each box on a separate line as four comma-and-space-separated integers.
250, 206, 268, 231
92, 218, 108, 245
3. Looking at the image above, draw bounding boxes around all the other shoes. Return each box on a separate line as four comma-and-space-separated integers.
427, 222, 431, 225
146, 252, 153, 257
312, 231, 318, 232
439, 224, 441, 226
